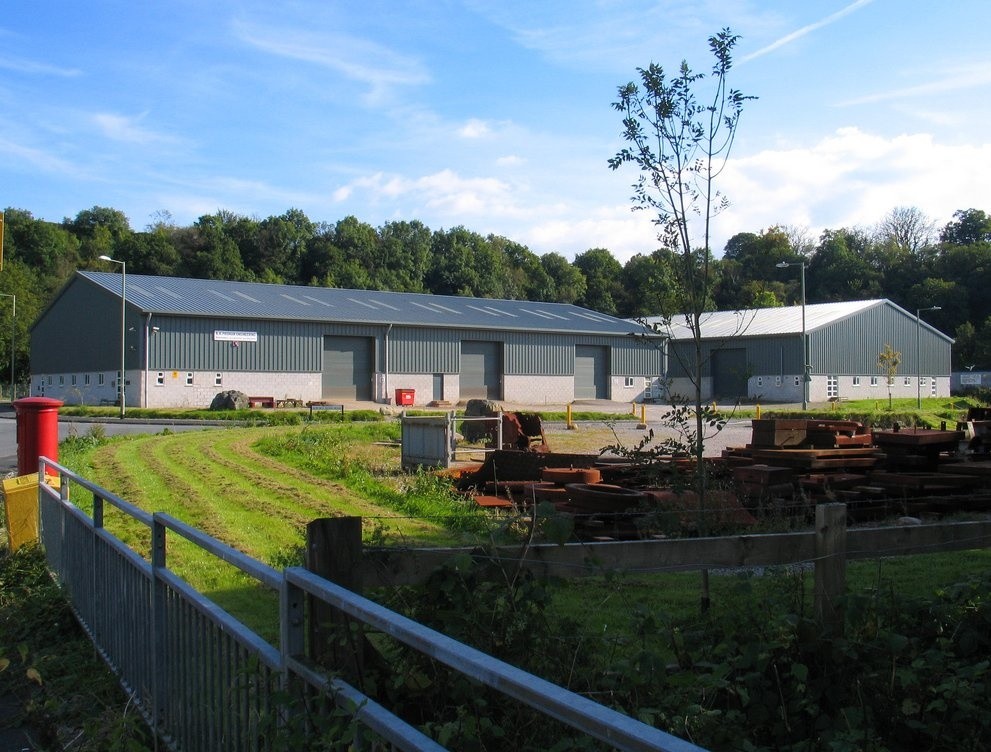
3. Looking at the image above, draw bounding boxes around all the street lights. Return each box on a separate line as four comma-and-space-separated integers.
775, 261, 814, 411
917, 305, 942, 409
97, 254, 126, 420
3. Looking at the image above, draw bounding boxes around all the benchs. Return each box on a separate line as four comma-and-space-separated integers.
249, 397, 275, 408
